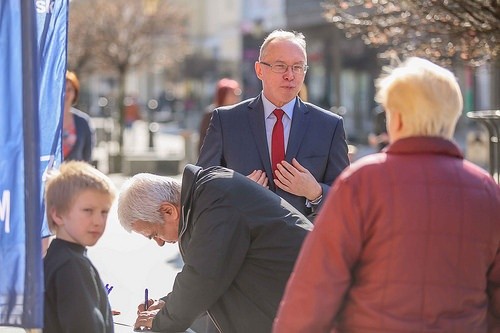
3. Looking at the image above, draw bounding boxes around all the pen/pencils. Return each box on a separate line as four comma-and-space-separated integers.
144, 289, 148, 311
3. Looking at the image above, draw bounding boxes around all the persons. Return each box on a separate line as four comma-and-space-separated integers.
61, 69, 91, 161
42, 160, 117, 333
272, 56, 500, 333
195, 29, 350, 222
115, 164, 315, 333
198, 79, 242, 156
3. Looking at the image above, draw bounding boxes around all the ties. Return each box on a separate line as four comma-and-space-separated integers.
272, 108, 285, 190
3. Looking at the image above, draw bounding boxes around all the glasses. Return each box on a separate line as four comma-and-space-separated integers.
260, 61, 309, 74
